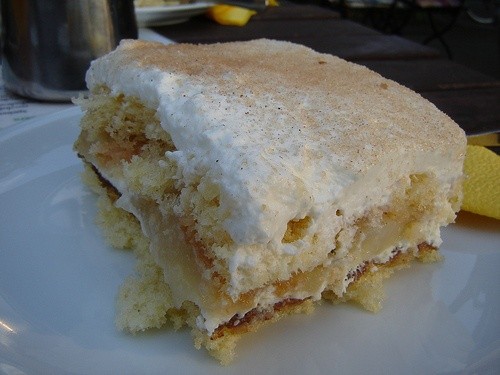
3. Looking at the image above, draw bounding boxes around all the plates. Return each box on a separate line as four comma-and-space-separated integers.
2, 102, 500, 375
135, 3, 215, 27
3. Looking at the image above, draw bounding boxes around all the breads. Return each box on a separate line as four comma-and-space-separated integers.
71, 39, 466, 364
